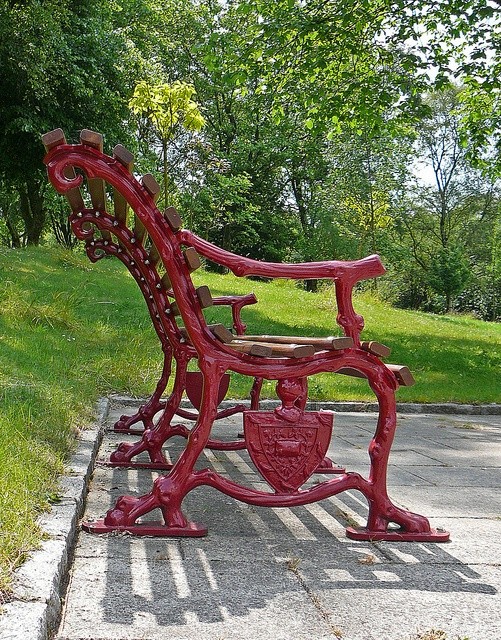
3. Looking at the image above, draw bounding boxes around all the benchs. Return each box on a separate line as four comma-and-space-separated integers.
39, 129, 449, 545
